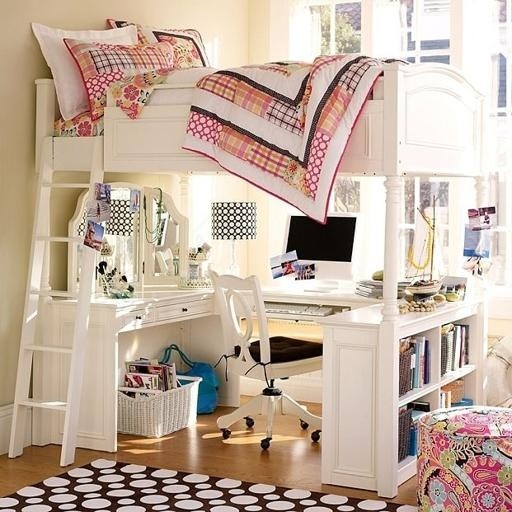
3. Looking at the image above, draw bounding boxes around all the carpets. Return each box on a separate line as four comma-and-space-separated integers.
0, 458, 418, 512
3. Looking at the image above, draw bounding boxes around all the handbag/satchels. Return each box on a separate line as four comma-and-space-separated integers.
177, 361, 218, 414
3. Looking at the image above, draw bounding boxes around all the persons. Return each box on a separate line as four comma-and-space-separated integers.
482, 211, 489, 225
96, 183, 103, 200
104, 184, 111, 202
280, 261, 315, 280
96, 203, 101, 221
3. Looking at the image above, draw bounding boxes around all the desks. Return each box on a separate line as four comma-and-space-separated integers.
36, 277, 383, 453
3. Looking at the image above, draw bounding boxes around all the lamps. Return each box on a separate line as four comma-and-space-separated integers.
210, 201, 257, 277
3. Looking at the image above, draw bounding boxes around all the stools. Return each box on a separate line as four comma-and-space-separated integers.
416, 406, 512, 512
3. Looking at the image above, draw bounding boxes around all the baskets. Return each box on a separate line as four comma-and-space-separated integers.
399, 407, 412, 464
118, 374, 203, 439
399, 347, 411, 396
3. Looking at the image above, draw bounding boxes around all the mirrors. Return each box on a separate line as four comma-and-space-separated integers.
67, 183, 189, 293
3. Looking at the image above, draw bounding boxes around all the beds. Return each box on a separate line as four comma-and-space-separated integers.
35, 53, 486, 319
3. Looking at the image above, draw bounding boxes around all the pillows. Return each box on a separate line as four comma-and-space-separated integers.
32, 22, 139, 122
107, 18, 210, 68
62, 38, 205, 122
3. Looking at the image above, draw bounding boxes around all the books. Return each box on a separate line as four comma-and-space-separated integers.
398, 399, 431, 465
440, 389, 452, 408
121, 357, 178, 400
355, 278, 410, 300
397, 323, 471, 397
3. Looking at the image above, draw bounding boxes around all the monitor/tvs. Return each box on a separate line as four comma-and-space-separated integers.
284, 214, 357, 289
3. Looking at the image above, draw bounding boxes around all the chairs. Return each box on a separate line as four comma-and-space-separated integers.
206, 265, 323, 450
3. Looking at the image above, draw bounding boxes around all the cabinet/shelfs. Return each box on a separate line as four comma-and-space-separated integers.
316, 301, 487, 500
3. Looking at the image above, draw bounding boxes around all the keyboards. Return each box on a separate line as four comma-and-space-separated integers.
265, 303, 334, 317
